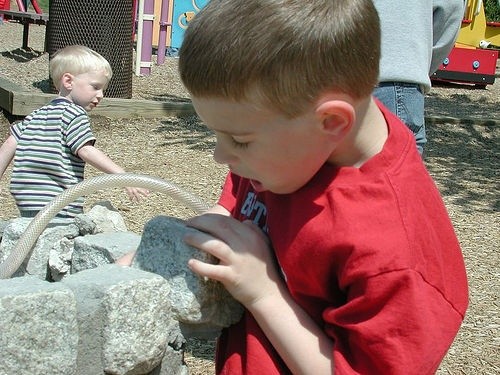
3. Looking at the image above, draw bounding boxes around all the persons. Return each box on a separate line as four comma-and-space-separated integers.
0, 44, 154, 224
107, 1, 470, 375
368, 1, 467, 169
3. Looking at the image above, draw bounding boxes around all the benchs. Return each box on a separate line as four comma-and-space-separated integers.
0, 9, 48, 50
